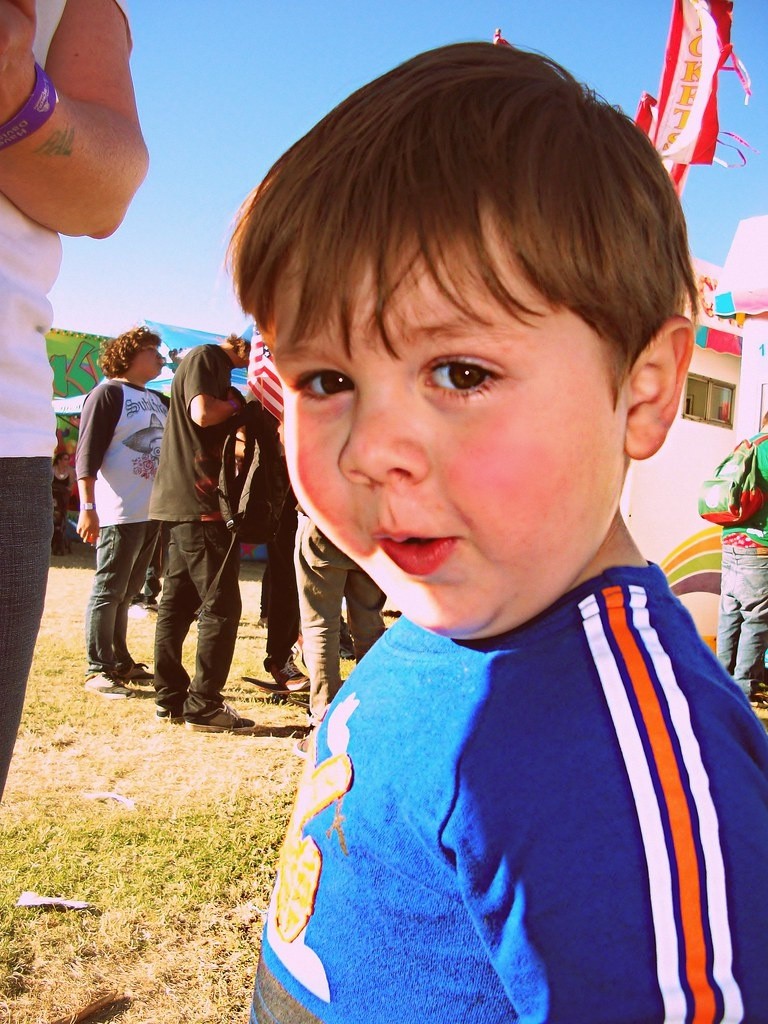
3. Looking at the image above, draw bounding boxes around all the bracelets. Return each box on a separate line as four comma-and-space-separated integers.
0, 63, 58, 151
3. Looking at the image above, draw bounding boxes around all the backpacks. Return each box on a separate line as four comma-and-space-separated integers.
697, 435, 767, 527
218, 411, 294, 545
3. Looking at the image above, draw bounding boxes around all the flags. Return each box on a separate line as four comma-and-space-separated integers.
656, 0, 760, 167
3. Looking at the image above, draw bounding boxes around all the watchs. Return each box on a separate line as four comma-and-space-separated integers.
80, 503, 96, 510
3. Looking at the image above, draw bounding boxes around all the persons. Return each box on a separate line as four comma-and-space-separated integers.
220, 34, 766, 1024
266, 452, 312, 692
150, 336, 284, 734
131, 535, 164, 613
75, 327, 189, 700
292, 491, 386, 762
0, 2, 150, 803
258, 564, 269, 629
698, 411, 768, 706
50, 452, 77, 556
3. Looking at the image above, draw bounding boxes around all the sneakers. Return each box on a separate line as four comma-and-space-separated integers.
83, 672, 133, 699
113, 662, 160, 684
271, 659, 311, 690
155, 701, 256, 731
